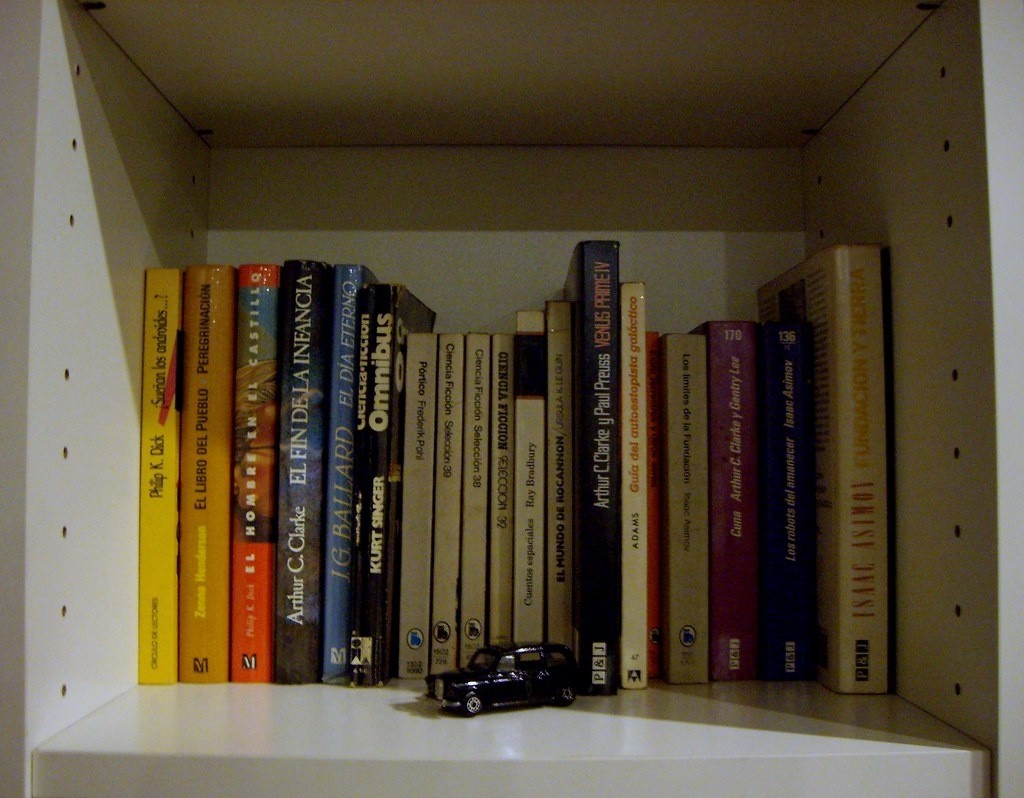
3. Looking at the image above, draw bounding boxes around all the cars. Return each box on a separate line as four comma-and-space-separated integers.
425, 641, 580, 716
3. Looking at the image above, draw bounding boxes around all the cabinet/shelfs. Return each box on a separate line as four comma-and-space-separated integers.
0, 0, 1024, 798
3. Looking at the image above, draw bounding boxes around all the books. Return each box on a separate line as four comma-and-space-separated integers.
137, 241, 891, 697
